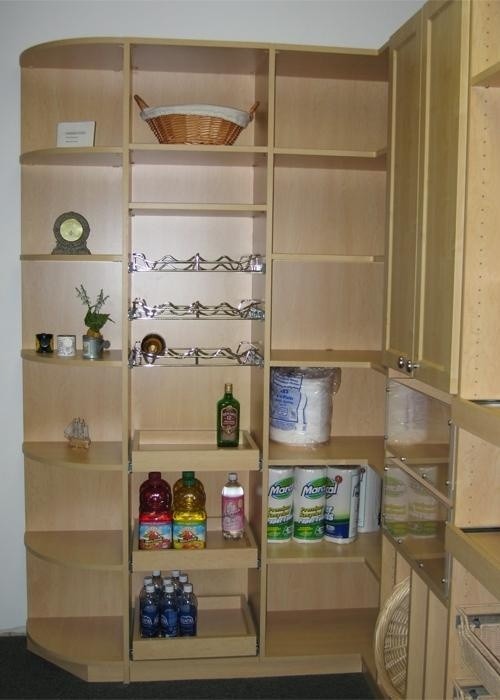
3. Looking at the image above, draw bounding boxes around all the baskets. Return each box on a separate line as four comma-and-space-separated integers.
135, 93, 261, 145
374, 577, 410, 700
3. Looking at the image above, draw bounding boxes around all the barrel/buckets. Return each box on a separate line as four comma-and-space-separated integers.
269, 367, 334, 447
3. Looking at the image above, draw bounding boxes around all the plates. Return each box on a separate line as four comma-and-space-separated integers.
141, 333, 166, 356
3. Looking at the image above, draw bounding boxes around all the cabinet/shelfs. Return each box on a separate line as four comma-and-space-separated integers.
383, 0, 500, 389
382, 380, 498, 700
18, 36, 385, 682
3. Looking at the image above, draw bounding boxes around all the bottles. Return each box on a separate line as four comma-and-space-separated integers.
215, 383, 241, 447
138, 569, 199, 640
136, 471, 208, 552
220, 472, 246, 544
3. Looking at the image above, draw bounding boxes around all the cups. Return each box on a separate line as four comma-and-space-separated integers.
82, 334, 111, 360
56, 334, 77, 357
35, 333, 54, 353
